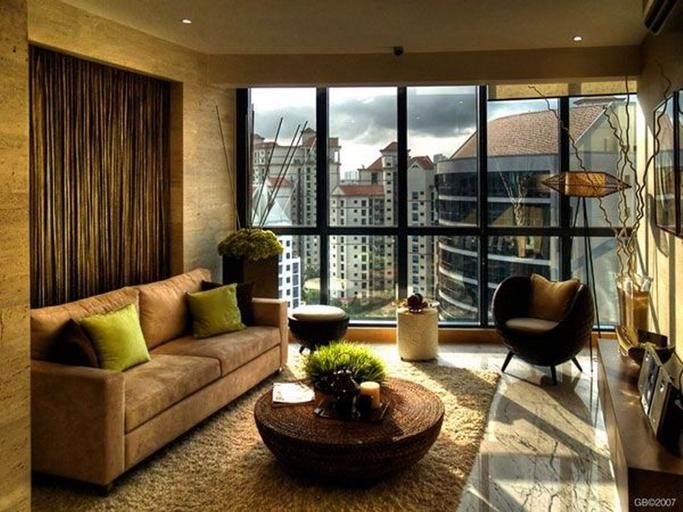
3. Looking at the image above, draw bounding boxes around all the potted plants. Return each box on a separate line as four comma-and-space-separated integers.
215, 227, 283, 298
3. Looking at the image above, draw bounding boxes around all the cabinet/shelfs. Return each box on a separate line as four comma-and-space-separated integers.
597, 337, 683, 511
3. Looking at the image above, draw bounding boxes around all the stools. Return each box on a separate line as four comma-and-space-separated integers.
286, 305, 351, 354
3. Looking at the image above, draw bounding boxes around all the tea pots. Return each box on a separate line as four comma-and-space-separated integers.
326, 366, 360, 421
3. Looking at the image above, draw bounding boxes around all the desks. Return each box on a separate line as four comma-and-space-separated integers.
396, 308, 438, 362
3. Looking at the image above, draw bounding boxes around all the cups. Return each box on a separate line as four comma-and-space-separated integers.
360, 380, 381, 408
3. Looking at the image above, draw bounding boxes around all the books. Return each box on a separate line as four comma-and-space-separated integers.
270, 381, 315, 407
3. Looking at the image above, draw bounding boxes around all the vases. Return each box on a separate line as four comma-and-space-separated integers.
616, 276, 653, 357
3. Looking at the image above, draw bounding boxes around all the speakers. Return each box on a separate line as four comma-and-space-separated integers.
637, 346, 662, 395
648, 365, 683, 438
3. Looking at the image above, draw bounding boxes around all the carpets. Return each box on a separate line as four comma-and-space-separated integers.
31, 364, 500, 512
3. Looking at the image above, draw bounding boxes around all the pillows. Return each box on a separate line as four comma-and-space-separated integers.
44, 301, 151, 371
530, 273, 579, 322
182, 277, 256, 340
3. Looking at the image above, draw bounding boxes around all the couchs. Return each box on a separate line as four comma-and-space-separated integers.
29, 268, 289, 499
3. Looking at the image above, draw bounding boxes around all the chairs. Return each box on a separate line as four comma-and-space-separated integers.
491, 273, 595, 384
